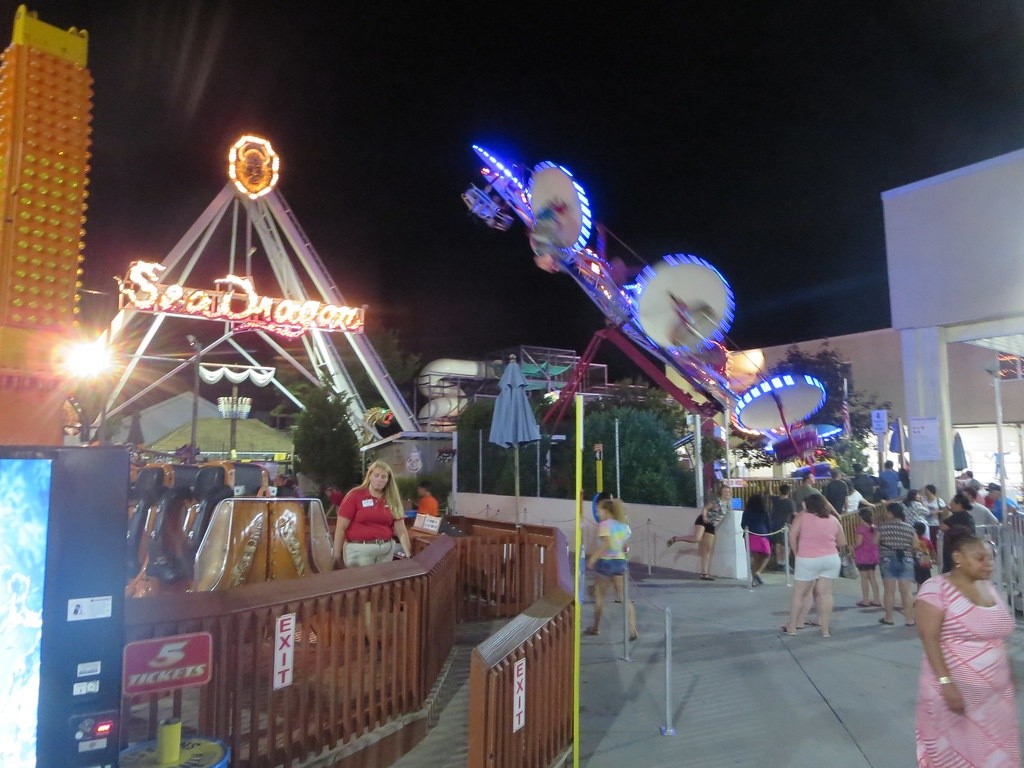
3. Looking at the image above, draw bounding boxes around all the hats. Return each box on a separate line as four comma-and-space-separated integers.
985, 483, 1001, 492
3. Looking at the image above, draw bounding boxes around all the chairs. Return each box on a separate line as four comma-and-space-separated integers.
126, 460, 343, 598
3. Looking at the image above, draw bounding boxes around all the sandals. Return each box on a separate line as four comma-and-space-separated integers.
666, 536, 676, 548
699, 573, 715, 580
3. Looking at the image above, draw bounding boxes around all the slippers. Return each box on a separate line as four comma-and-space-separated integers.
855, 600, 870, 607
822, 631, 830, 638
783, 627, 798, 635
878, 617, 895, 625
781, 622, 805, 629
906, 619, 915, 626
809, 619, 822, 626
869, 601, 882, 606
614, 597, 634, 603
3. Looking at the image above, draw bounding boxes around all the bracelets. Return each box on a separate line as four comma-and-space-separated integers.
406, 556, 413, 558
937, 675, 952, 684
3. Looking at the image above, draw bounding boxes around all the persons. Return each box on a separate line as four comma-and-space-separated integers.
914, 530, 1021, 768
332, 460, 414, 650
992, 448, 1012, 480
273, 474, 305, 509
416, 480, 439, 516
902, 470, 1021, 540
780, 493, 848, 638
668, 485, 732, 581
587, 498, 639, 641
773, 460, 898, 570
740, 495, 776, 587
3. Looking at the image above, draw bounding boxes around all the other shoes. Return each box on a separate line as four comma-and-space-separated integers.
588, 584, 596, 602
753, 572, 763, 583
752, 581, 757, 587
587, 625, 600, 635
628, 628, 638, 642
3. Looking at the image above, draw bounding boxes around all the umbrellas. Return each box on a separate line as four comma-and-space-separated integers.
488, 354, 542, 524
954, 432, 968, 472
888, 416, 910, 453
